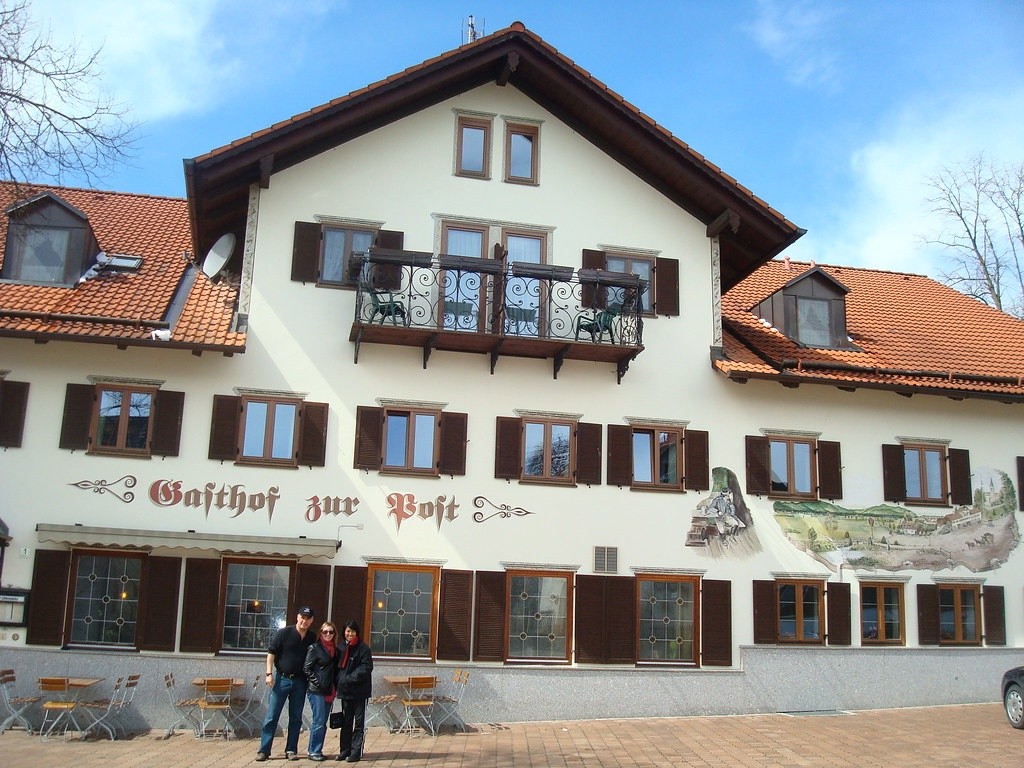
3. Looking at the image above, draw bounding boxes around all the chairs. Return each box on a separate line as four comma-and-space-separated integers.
366, 669, 469, 734
0, 667, 141, 742
365, 281, 405, 324
575, 301, 621, 344
163, 672, 270, 740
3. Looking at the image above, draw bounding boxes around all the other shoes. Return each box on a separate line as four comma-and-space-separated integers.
335, 753, 351, 761
286, 751, 298, 761
346, 755, 361, 762
255, 753, 269, 761
307, 754, 326, 761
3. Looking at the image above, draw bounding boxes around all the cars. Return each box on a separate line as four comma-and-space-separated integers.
1001, 665, 1024, 731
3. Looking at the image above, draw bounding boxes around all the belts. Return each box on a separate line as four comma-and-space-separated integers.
279, 671, 303, 679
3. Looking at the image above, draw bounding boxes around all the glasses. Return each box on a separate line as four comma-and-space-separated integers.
321, 630, 334, 634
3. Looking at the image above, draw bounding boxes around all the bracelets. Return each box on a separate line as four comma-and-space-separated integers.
266, 672, 273, 677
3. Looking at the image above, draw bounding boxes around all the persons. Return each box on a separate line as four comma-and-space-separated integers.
255, 607, 316, 761
336, 621, 374, 762
303, 621, 342, 761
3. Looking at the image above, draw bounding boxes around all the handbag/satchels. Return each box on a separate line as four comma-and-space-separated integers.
329, 712, 345, 729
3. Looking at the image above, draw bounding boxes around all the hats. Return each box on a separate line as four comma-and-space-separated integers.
297, 606, 314, 618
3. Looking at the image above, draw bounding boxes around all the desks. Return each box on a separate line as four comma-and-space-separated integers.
34, 676, 101, 742
193, 677, 246, 742
382, 675, 443, 738
